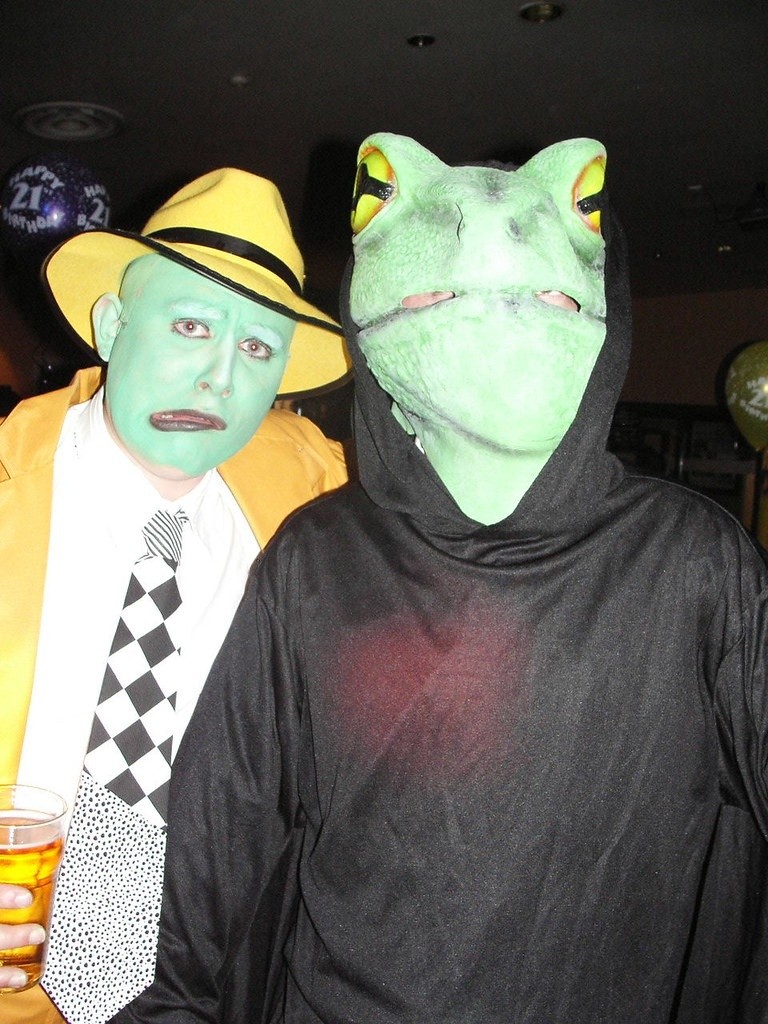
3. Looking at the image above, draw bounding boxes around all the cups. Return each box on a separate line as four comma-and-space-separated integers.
0, 782, 68, 994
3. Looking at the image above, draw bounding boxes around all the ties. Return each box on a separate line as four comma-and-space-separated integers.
35, 509, 187, 1024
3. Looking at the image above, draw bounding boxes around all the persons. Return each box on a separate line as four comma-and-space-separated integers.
112, 130, 768, 1024
0, 164, 354, 1024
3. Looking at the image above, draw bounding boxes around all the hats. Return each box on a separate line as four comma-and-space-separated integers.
38, 166, 351, 401
